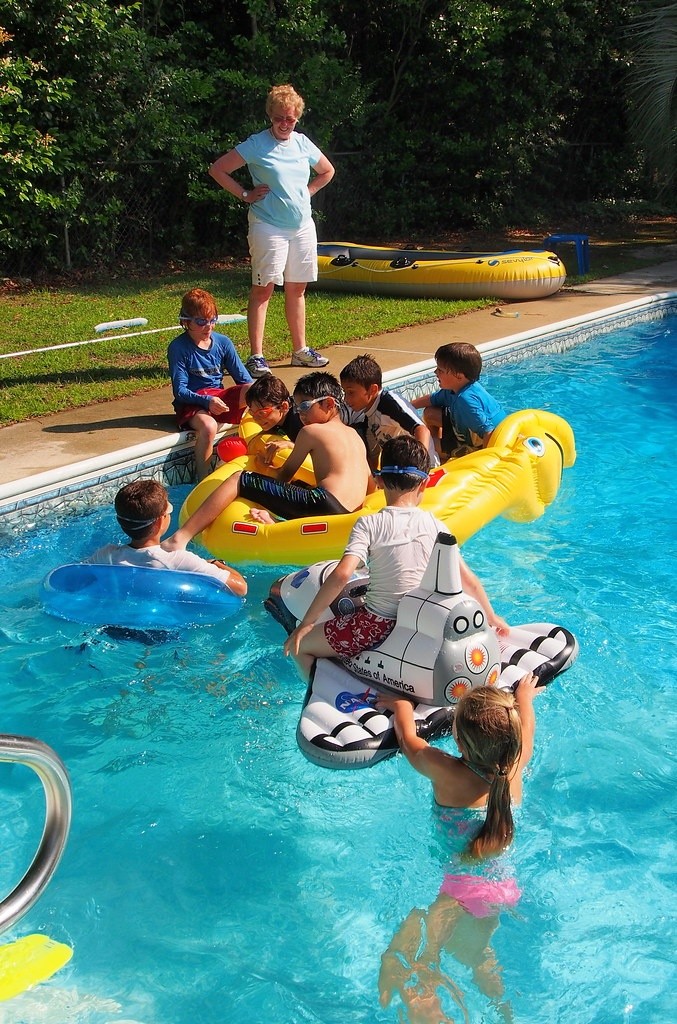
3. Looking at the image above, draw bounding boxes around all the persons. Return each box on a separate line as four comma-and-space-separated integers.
165, 288, 261, 482
155, 370, 380, 554
207, 83, 336, 379
407, 342, 509, 466
280, 432, 511, 684
78, 476, 250, 598
212, 372, 366, 471
375, 671, 546, 861
338, 352, 441, 490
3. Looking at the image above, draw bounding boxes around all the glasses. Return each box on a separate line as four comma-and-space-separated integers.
271, 116, 298, 123
178, 316, 219, 326
115, 500, 174, 531
293, 395, 341, 413
249, 402, 282, 420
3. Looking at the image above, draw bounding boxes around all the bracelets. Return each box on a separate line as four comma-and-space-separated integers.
210, 558, 226, 567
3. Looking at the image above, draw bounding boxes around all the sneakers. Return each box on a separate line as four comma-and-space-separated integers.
290, 347, 330, 368
245, 354, 273, 379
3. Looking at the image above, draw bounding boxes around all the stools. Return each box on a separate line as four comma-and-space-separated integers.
543, 232, 590, 277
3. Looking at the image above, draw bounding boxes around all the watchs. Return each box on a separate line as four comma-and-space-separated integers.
241, 189, 249, 201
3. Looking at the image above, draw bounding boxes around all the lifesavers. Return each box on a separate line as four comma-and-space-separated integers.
39, 564, 245, 629
